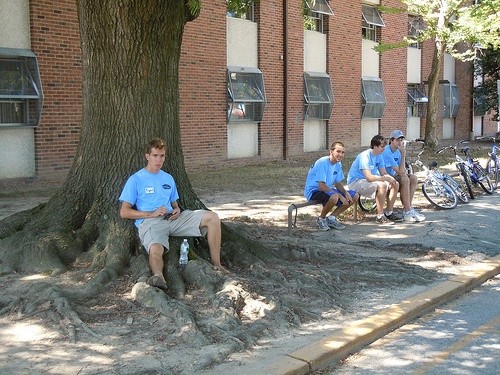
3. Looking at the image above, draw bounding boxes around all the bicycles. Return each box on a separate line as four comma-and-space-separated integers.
436, 137, 494, 199
484, 137, 500, 188
410, 139, 469, 209
358, 192, 377, 211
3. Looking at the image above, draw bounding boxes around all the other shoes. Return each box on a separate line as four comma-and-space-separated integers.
147, 275, 168, 290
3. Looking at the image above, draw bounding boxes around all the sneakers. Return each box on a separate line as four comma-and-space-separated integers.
376, 215, 394, 225
326, 217, 346, 230
384, 212, 403, 222
316, 217, 330, 231
403, 210, 426, 221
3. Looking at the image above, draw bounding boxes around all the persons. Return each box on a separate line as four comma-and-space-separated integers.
118, 138, 230, 291
303, 141, 359, 231
347, 135, 404, 225
381, 130, 426, 223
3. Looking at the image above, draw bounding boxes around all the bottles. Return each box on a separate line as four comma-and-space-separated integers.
179, 239, 190, 265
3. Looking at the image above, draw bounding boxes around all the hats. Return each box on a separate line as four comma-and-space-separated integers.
390, 130, 405, 139
